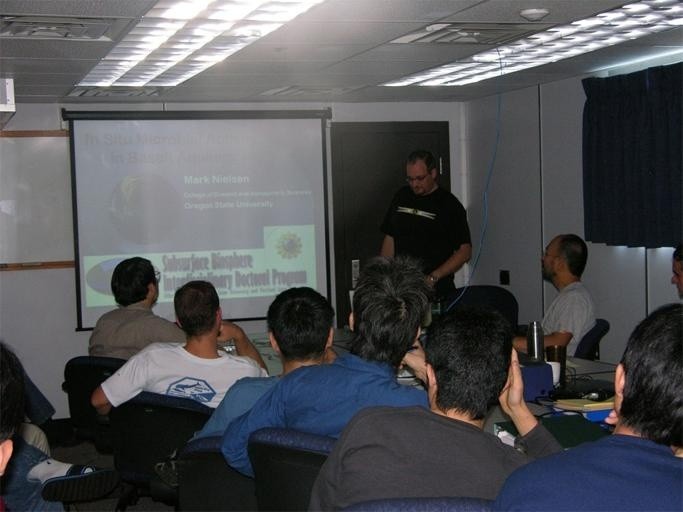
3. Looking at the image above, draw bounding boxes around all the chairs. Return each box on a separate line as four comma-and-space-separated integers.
61, 356, 130, 431
176, 435, 257, 512
115, 393, 216, 512
248, 426, 337, 512
575, 320, 610, 360
342, 497, 495, 512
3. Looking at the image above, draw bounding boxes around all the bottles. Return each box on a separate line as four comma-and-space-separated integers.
526, 322, 544, 362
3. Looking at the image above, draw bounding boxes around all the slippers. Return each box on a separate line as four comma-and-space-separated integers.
41, 462, 114, 504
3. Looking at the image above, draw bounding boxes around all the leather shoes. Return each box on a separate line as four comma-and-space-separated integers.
45, 421, 75, 444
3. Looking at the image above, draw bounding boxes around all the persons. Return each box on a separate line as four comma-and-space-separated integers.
1, 236, 683, 511
379, 150, 472, 312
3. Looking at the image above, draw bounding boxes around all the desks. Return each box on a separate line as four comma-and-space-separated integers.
217, 328, 623, 458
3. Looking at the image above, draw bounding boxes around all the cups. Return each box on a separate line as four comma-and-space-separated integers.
545, 346, 567, 371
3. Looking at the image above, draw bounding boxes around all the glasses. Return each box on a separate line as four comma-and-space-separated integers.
405, 173, 429, 182
541, 251, 567, 258
154, 270, 160, 282
